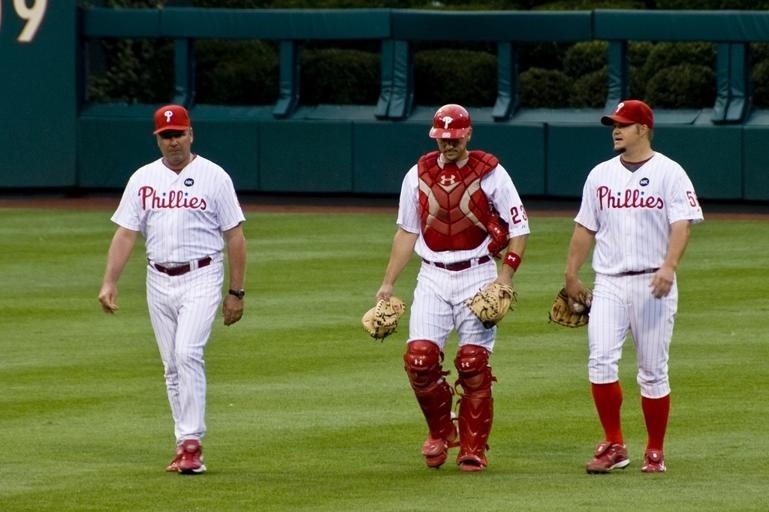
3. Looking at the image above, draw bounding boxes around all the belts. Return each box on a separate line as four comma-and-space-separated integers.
144, 254, 212, 278
421, 253, 495, 272
610, 267, 663, 279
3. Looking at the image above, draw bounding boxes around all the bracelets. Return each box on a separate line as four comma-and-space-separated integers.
502, 252, 522, 270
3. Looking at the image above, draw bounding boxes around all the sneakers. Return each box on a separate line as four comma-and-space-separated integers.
583, 440, 632, 475
456, 440, 490, 471
177, 439, 209, 475
421, 421, 458, 469
640, 445, 668, 473
163, 444, 184, 472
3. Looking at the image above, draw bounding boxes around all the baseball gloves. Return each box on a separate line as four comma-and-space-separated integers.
361, 296, 406, 339
466, 284, 515, 329
548, 280, 593, 326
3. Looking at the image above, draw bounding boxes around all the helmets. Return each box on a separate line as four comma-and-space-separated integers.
426, 102, 474, 140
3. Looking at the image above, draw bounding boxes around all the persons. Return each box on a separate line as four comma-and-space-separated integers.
565, 101, 704, 473
98, 105, 246, 474
375, 105, 530, 471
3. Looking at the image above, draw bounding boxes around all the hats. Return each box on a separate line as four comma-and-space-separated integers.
151, 103, 193, 135
599, 98, 656, 130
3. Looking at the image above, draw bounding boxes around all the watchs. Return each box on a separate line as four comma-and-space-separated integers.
228, 289, 245, 300
